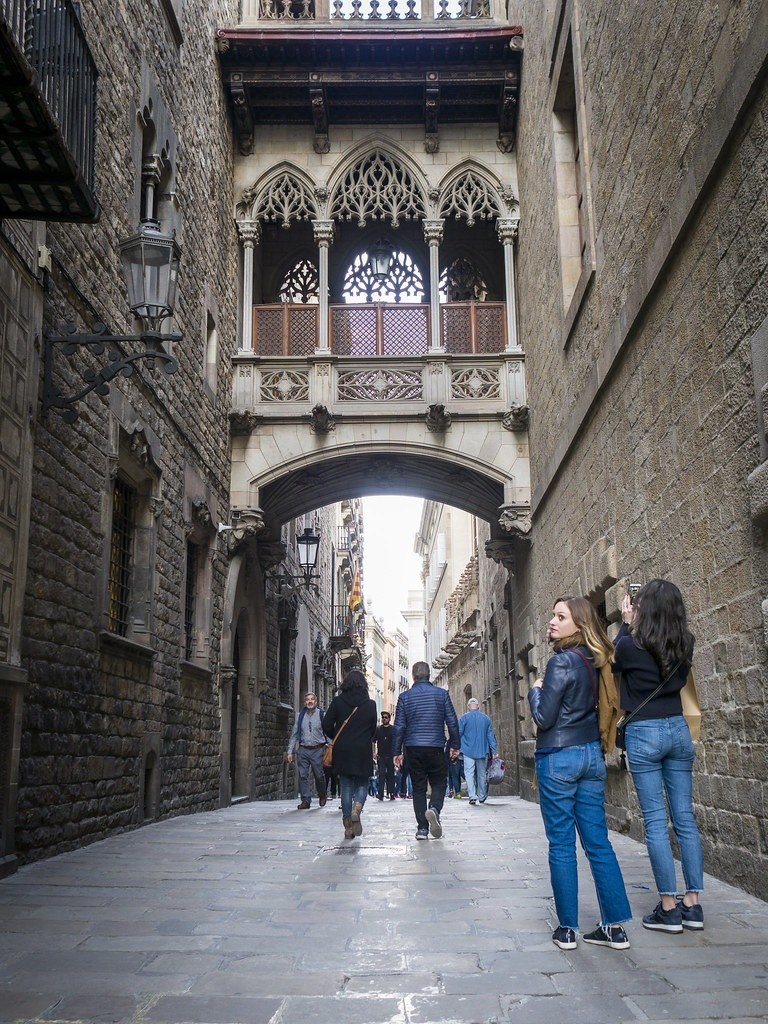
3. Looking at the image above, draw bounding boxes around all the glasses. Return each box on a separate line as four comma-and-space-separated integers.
382, 716, 388, 719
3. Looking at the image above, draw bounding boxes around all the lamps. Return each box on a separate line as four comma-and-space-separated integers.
266, 528, 321, 593
43, 222, 184, 414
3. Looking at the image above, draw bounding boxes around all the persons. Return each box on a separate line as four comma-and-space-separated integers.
287, 692, 332, 810
390, 662, 461, 840
371, 711, 396, 800
444, 736, 492, 800
611, 579, 705, 934
369, 751, 432, 799
321, 670, 377, 840
527, 596, 632, 950
458, 698, 498, 804
323, 767, 341, 798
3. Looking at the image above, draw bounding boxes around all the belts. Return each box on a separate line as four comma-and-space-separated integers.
299, 743, 325, 749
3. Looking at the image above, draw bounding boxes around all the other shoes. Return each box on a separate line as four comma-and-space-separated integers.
339, 805, 343, 809
369, 790, 462, 800
479, 793, 488, 802
469, 799, 476, 804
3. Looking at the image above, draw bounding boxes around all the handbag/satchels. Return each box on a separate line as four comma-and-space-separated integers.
321, 739, 333, 768
615, 714, 626, 751
486, 757, 505, 785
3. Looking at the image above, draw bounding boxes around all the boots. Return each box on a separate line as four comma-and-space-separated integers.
350, 802, 364, 836
343, 817, 355, 839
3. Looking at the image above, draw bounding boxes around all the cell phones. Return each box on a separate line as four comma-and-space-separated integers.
628, 584, 642, 605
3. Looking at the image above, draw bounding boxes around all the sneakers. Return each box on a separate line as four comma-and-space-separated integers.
320, 794, 326, 806
675, 894, 704, 929
642, 900, 683, 933
552, 925, 577, 950
582, 920, 630, 950
425, 806, 443, 838
415, 828, 429, 840
298, 800, 310, 809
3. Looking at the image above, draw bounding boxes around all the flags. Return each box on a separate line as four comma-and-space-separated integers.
349, 563, 361, 611
